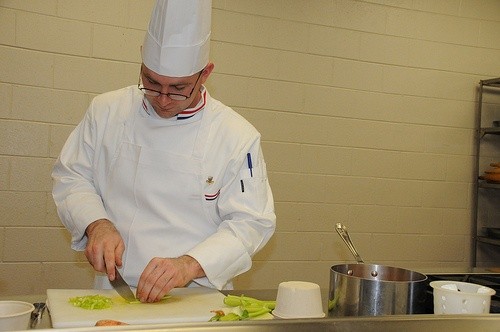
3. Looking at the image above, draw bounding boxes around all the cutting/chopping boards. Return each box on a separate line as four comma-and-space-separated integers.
44, 287, 272, 328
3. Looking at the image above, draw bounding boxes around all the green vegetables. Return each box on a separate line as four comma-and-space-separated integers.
207, 295, 276, 321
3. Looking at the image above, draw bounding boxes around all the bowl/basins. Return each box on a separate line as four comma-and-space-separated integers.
272, 280, 326, 319
429, 281, 496, 314
0, 301, 36, 331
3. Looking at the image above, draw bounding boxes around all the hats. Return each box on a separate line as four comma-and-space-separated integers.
140, 1, 211, 78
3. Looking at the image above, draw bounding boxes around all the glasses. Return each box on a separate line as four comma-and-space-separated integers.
138, 62, 205, 101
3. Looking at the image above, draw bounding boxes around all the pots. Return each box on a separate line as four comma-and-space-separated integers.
328, 222, 428, 316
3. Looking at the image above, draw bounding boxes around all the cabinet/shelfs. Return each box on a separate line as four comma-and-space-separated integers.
471, 74, 500, 271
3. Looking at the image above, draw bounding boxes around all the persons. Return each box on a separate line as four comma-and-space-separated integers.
50, 0, 276, 303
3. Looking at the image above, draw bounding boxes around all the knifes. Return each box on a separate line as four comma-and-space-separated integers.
105, 267, 135, 302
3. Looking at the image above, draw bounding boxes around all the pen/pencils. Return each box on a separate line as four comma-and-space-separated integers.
247, 153, 254, 178
